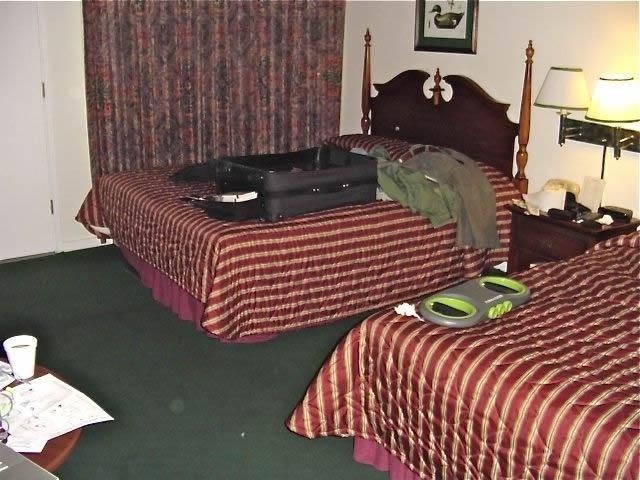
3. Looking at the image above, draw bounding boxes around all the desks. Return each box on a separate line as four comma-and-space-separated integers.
1, 357, 83, 475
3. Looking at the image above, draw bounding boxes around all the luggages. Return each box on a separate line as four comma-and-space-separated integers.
215, 146, 378, 223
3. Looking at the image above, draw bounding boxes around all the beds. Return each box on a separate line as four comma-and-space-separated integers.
285, 230, 640, 480
75, 27, 534, 345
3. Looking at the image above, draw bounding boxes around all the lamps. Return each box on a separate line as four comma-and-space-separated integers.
534, 67, 639, 160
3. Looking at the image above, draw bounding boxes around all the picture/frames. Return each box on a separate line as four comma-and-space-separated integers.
414, 0, 479, 55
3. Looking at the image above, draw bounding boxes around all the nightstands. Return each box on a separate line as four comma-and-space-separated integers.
504, 201, 640, 275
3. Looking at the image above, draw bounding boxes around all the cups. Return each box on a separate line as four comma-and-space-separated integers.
581, 176, 606, 214
3, 334, 38, 380
